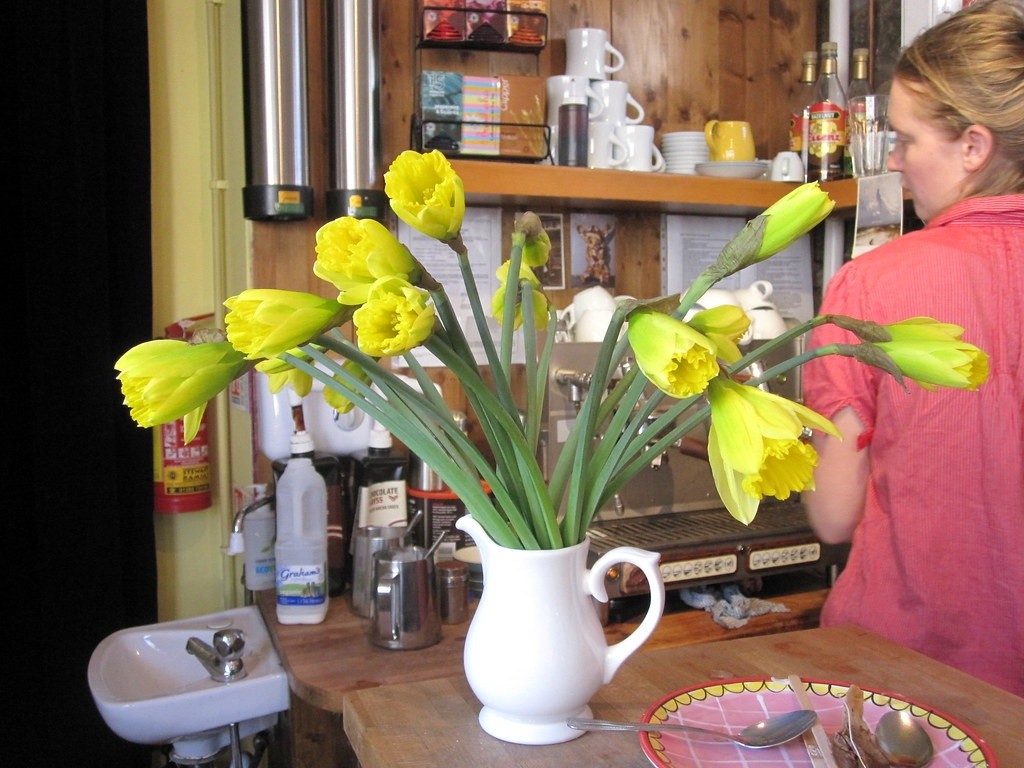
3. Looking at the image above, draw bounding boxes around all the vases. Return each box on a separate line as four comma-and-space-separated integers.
455, 514, 665, 745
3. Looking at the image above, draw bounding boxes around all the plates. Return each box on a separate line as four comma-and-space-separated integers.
659, 131, 710, 175
637, 675, 1002, 768
693, 161, 768, 179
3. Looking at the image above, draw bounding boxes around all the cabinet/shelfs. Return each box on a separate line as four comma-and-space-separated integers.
251, 0, 913, 768
409, 0, 555, 165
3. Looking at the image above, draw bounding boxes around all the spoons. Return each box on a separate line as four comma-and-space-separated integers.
874, 710, 934, 767
565, 710, 817, 749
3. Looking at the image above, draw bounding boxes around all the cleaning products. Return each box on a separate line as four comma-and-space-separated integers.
241, 480, 277, 591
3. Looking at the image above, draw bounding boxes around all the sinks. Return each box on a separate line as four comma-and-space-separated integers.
87, 611, 236, 743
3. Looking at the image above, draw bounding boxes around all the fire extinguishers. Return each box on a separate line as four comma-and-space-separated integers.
151, 310, 215, 515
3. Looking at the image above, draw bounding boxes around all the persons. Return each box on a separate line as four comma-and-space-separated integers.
800, 0, 1024, 698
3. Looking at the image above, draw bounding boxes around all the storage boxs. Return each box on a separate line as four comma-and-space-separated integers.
414, 69, 545, 160
414, 0, 545, 46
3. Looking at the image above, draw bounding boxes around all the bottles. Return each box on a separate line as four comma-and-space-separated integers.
433, 560, 471, 625
276, 451, 330, 625
788, 42, 873, 182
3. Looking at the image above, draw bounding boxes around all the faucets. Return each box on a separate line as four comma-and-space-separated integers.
184, 629, 248, 682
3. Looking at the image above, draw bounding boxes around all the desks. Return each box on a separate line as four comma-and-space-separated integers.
344, 623, 1024, 768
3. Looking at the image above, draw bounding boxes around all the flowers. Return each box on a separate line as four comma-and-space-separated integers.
114, 149, 989, 548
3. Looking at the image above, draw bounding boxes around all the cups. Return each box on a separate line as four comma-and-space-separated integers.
370, 546, 442, 652
704, 119, 756, 161
770, 150, 805, 183
559, 280, 791, 342
537, 27, 666, 174
351, 527, 414, 617
845, 93, 891, 178
